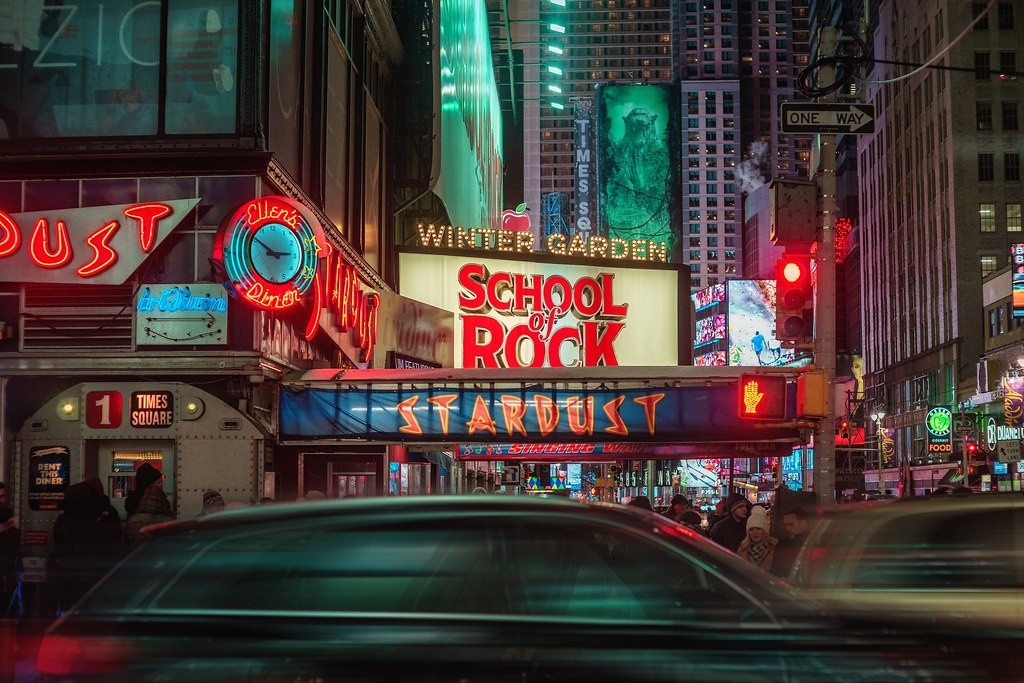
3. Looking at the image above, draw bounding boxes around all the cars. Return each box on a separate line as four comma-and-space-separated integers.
694, 508, 707, 519
933, 484, 973, 496
853, 490, 885, 501
31, 496, 981, 682
787, 494, 1024, 640
654, 505, 671, 513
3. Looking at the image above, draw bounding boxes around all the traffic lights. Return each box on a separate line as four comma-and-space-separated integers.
657, 496, 662, 503
841, 419, 849, 438
958, 467, 962, 475
969, 444, 975, 455
849, 420, 857, 436
736, 372, 788, 422
592, 487, 596, 495
967, 466, 975, 475
775, 253, 812, 341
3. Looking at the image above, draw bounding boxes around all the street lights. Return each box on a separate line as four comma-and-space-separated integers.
870, 403, 885, 498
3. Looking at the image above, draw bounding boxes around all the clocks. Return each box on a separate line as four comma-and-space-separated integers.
249, 222, 304, 284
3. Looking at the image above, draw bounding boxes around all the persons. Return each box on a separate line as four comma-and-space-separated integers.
710, 493, 750, 553
750, 330, 781, 365
660, 495, 689, 523
677, 512, 706, 537
708, 496, 727, 532
0, 482, 21, 616
737, 506, 780, 568
53, 464, 227, 611
775, 510, 810, 576
472, 488, 486, 495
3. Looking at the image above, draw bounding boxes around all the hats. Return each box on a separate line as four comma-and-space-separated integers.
203, 489, 224, 508
722, 493, 752, 512
136, 463, 163, 491
631, 496, 654, 513
671, 494, 688, 505
746, 505, 770, 536
681, 511, 702, 525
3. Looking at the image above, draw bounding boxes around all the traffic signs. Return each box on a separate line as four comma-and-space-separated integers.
781, 103, 877, 134
